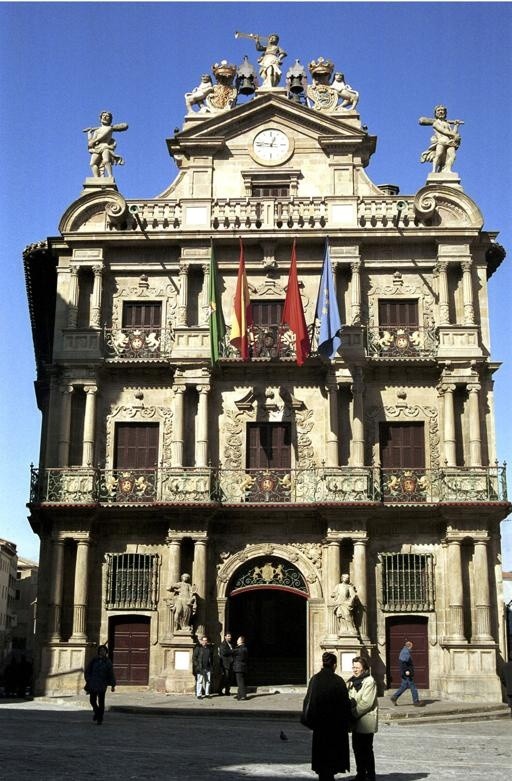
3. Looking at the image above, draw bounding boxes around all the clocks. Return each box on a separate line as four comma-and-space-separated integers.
247, 126, 296, 168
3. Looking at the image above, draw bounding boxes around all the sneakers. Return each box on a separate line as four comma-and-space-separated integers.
414, 701, 425, 707
93, 714, 102, 725
349, 775, 367, 781
390, 697, 398, 706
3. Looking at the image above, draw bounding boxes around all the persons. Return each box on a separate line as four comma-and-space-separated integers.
218, 632, 234, 695
85, 645, 116, 724
1, 658, 19, 699
230, 635, 249, 700
254, 32, 286, 87
390, 641, 425, 707
85, 112, 125, 176
165, 572, 194, 632
343, 656, 379, 781
420, 103, 460, 177
191, 635, 212, 699
17, 655, 34, 696
331, 574, 358, 633
300, 653, 354, 781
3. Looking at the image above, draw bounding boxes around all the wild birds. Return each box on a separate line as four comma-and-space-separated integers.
280, 731, 288, 740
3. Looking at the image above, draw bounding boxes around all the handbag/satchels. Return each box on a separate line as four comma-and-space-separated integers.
300, 711, 313, 730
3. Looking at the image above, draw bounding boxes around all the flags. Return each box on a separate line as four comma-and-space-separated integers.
206, 236, 226, 368
313, 236, 343, 364
282, 236, 311, 368
228, 237, 255, 359
